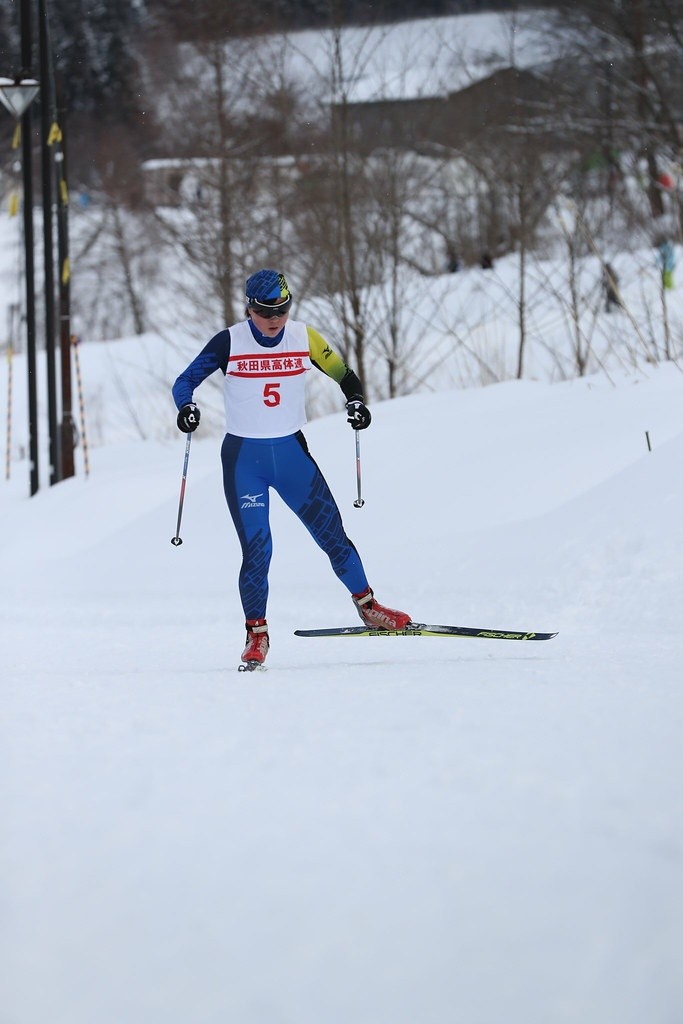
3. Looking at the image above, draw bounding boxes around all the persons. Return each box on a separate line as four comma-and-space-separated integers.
171, 269, 411, 671
601, 263, 620, 312
657, 244, 673, 289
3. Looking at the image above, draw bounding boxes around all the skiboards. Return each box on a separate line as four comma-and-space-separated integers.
237, 620, 560, 672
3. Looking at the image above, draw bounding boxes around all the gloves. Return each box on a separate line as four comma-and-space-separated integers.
177, 403, 201, 433
347, 404, 372, 431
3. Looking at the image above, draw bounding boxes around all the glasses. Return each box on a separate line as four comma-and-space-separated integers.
251, 295, 293, 319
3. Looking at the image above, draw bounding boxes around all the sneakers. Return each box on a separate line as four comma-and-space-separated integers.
241, 620, 269, 663
351, 586, 411, 631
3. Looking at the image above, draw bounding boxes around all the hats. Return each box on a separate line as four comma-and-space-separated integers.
246, 270, 291, 309
